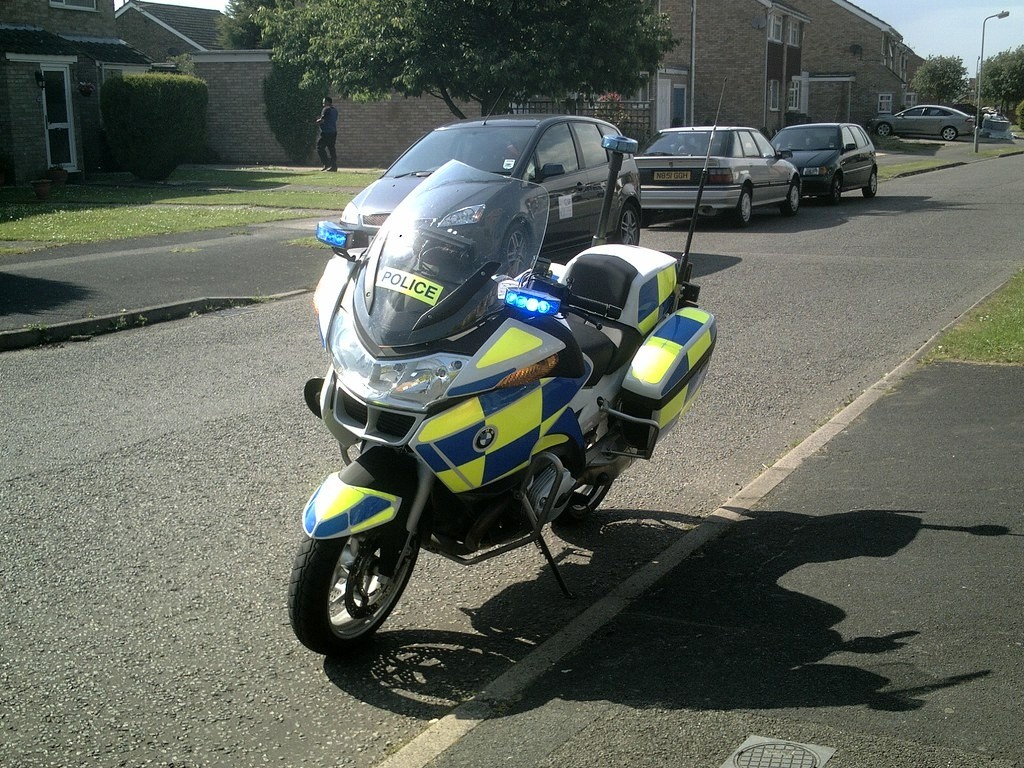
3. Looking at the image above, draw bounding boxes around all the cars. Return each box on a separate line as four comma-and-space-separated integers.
770, 123, 878, 206
866, 104, 975, 142
337, 87, 641, 272
631, 124, 801, 230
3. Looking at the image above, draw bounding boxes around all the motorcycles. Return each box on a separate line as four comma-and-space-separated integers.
287, 75, 728, 654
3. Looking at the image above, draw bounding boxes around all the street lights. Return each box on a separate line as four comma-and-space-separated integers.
973, 10, 1010, 153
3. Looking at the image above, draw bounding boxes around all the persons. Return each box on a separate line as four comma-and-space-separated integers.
315, 97, 338, 172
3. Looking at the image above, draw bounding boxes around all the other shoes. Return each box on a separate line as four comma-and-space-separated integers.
321, 165, 337, 172
